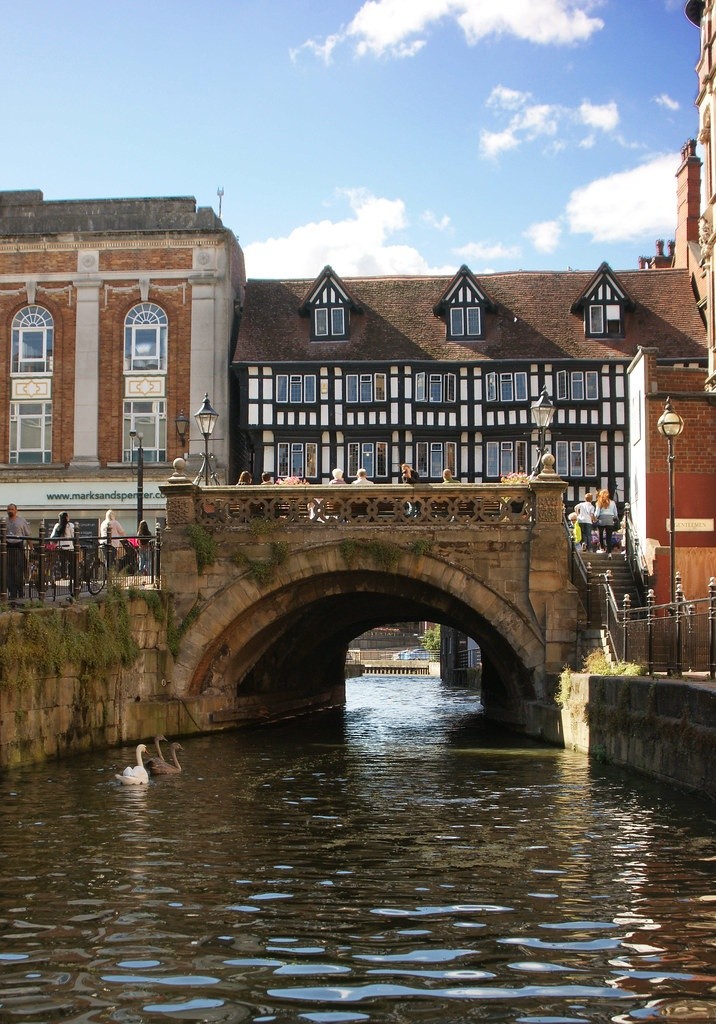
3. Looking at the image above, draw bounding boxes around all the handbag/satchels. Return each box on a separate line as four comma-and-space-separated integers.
592, 518, 599, 529
574, 520, 582, 544
612, 517, 621, 532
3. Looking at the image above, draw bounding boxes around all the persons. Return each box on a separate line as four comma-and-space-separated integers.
574, 493, 598, 553
136, 520, 151, 576
260, 472, 272, 485
398, 464, 419, 484
98, 509, 125, 579
351, 468, 374, 484
329, 469, 346, 484
50, 511, 74, 579
443, 468, 460, 483
593, 489, 617, 560
5, 503, 33, 608
236, 471, 252, 485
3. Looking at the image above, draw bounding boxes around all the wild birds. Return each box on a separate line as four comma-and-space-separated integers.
144, 734, 167, 767
146, 742, 184, 775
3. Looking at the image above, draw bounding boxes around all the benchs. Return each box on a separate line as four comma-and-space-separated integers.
226, 498, 501, 522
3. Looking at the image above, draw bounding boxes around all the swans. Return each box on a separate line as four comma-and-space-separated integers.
115, 744, 149, 785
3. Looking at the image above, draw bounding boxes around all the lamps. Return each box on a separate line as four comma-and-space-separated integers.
175, 409, 189, 447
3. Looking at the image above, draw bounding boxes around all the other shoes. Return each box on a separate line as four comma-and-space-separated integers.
582, 543, 586, 551
608, 554, 612, 560
596, 549, 605, 554
589, 550, 593, 553
9, 593, 17, 598
19, 591, 23, 598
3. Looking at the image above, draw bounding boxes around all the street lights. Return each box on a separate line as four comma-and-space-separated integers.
656, 394, 685, 618
530, 384, 557, 476
129, 429, 144, 534
192, 392, 223, 486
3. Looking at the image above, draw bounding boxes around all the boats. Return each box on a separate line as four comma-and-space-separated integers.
393, 648, 433, 660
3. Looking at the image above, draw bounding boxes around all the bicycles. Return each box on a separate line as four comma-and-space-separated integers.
28, 542, 107, 603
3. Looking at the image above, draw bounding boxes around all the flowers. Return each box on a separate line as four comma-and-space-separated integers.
274, 477, 310, 485
499, 470, 532, 485
